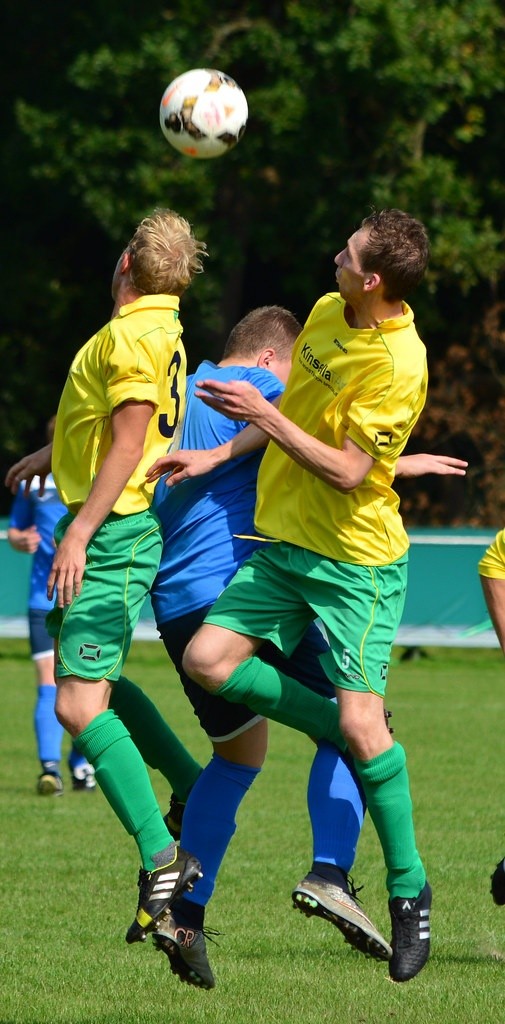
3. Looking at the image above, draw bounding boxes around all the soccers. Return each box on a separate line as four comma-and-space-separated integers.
158, 66, 251, 161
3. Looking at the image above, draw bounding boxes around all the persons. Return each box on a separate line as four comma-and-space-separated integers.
478, 527, 505, 907
7, 204, 469, 991
7, 415, 96, 795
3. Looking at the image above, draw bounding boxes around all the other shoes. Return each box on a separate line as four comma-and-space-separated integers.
491, 859, 505, 905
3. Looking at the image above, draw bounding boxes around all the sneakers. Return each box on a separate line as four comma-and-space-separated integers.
388, 881, 432, 982
153, 912, 224, 991
125, 845, 204, 944
292, 872, 393, 962
69, 759, 96, 791
38, 771, 63, 794
